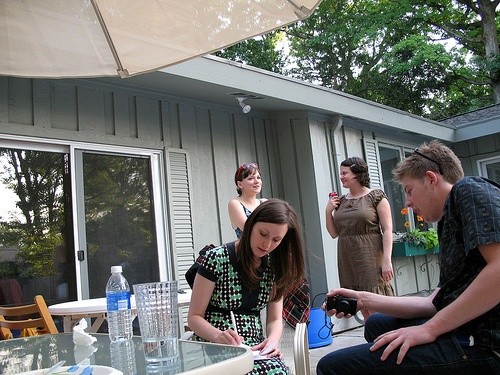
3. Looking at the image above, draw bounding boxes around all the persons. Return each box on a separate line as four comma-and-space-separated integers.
325, 157, 396, 319
188, 198, 305, 375
228, 163, 270, 242
316, 139, 500, 375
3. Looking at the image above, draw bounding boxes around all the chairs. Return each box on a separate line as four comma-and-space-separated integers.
0, 295, 59, 340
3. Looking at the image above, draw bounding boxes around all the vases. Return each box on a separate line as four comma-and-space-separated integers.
391, 238, 440, 258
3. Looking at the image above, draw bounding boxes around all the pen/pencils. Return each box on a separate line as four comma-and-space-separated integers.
228, 310, 240, 337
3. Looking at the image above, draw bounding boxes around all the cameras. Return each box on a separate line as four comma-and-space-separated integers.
327, 294, 357, 315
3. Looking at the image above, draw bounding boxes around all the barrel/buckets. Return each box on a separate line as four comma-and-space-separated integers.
308, 308, 332, 349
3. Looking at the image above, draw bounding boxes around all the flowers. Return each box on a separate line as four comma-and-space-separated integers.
393, 208, 439, 253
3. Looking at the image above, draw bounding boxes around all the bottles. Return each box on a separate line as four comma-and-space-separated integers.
106, 266, 133, 343
110, 343, 137, 375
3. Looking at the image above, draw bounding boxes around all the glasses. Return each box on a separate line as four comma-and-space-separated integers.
237, 163, 258, 178
412, 148, 444, 175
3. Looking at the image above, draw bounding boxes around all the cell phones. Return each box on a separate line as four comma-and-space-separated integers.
329, 192, 339, 209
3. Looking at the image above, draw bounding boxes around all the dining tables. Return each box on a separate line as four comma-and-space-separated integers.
0, 332, 254, 375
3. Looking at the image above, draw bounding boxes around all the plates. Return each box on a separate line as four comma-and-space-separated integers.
13, 364, 123, 375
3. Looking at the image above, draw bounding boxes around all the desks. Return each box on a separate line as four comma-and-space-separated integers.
47, 289, 195, 340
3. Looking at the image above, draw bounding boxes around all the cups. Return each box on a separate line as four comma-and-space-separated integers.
131, 282, 178, 342
143, 342, 182, 375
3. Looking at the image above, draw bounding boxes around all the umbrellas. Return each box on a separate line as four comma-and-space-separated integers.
0, 0, 324, 79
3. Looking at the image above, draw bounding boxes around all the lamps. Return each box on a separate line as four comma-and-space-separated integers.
235, 97, 251, 114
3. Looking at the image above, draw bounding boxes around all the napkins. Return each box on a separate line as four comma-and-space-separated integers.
71, 318, 97, 346
